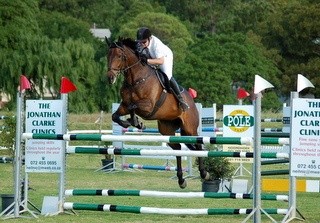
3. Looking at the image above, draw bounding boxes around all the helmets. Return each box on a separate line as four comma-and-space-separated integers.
135, 27, 151, 43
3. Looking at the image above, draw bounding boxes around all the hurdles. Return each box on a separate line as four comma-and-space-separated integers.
0, 90, 305, 221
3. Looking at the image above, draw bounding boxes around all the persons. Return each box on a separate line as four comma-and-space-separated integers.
137, 28, 190, 110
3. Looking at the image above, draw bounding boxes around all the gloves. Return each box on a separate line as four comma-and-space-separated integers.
140, 56, 147, 65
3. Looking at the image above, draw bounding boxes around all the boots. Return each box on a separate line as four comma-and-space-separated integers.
169, 77, 187, 110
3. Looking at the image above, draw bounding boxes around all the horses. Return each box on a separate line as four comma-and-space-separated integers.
104, 35, 210, 189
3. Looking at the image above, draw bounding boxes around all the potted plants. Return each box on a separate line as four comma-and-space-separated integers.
0, 113, 25, 215
98, 141, 113, 170
194, 144, 235, 193
